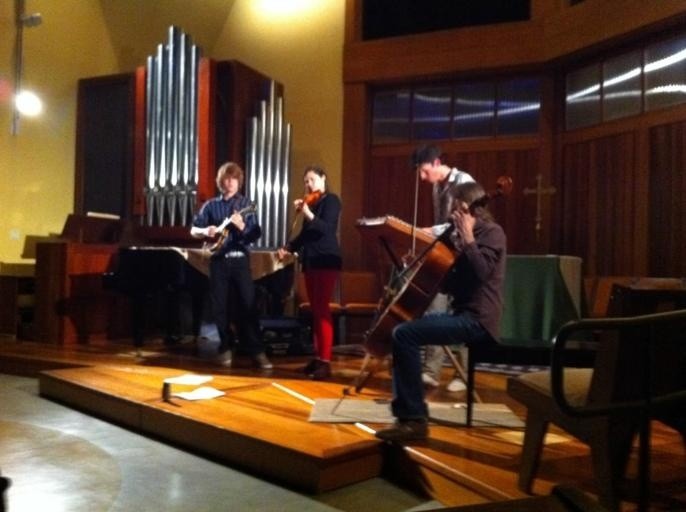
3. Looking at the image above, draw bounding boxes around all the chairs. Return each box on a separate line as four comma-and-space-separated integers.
503, 278, 685, 491
296, 270, 396, 350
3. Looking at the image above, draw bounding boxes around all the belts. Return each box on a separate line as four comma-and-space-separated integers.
226, 251, 243, 257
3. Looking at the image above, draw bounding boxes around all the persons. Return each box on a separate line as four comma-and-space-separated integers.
189, 161, 276, 371
410, 141, 478, 392
273, 166, 343, 382
375, 181, 509, 444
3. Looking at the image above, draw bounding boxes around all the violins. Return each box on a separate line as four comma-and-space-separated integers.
296, 190, 322, 212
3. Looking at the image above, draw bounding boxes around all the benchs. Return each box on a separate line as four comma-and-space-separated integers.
465, 334, 599, 430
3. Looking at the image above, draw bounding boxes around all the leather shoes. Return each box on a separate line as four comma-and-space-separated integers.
374, 422, 429, 442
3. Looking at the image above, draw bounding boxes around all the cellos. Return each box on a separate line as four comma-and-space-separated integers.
362, 176, 514, 361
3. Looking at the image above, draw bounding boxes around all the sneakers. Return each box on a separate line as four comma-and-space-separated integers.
219, 350, 231, 366
301, 360, 318, 375
249, 351, 272, 370
310, 362, 332, 379
422, 373, 439, 385
447, 380, 467, 392
